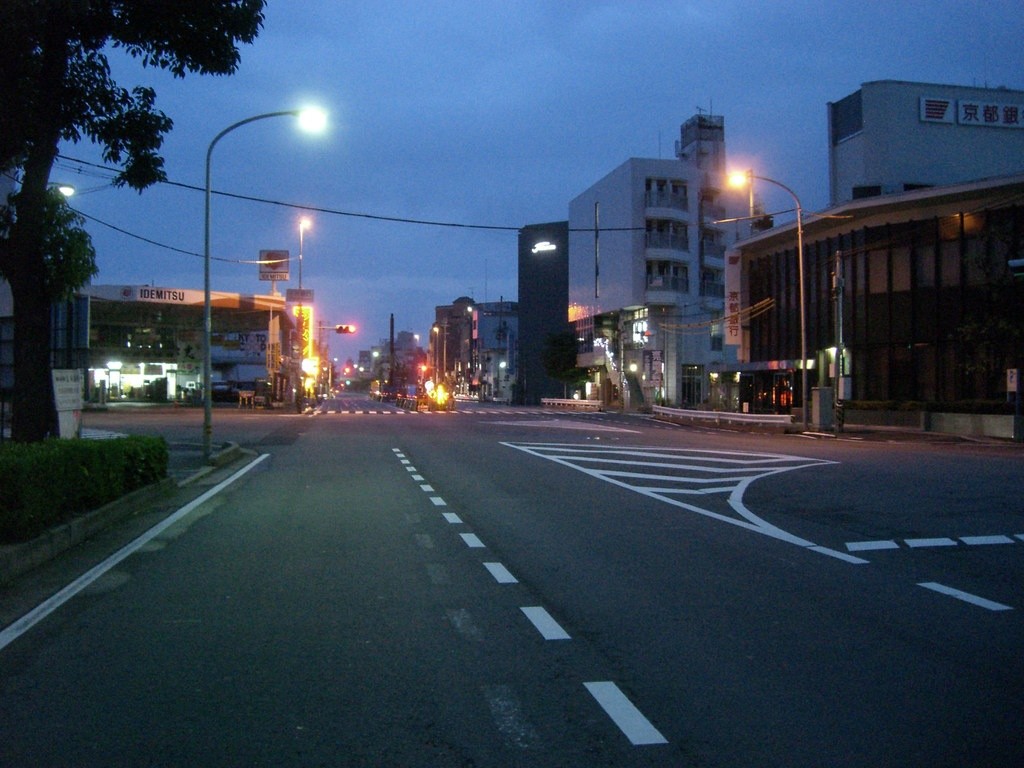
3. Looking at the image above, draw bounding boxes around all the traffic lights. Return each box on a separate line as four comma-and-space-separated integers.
334, 325, 357, 333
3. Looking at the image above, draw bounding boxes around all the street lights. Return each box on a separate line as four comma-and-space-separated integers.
432, 326, 439, 386
729, 171, 809, 434
296, 218, 312, 415
202, 106, 328, 455
